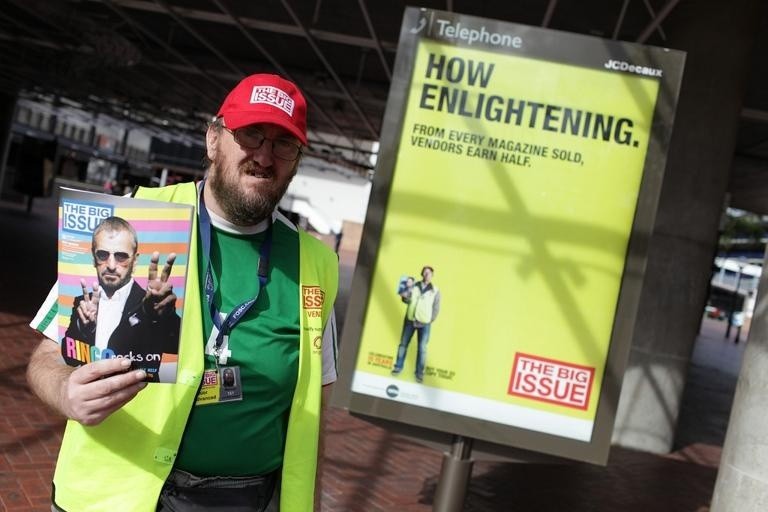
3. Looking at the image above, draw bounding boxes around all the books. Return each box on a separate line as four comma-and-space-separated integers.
55, 183, 197, 387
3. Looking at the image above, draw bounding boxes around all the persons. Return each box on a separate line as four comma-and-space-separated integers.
59, 214, 184, 385
219, 367, 236, 387
389, 263, 440, 385
22, 69, 343, 512
398, 277, 414, 298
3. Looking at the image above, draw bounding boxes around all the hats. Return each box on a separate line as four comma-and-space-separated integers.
215, 73, 309, 147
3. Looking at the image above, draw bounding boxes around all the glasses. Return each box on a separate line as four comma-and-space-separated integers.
219, 123, 301, 161
95, 249, 131, 262
